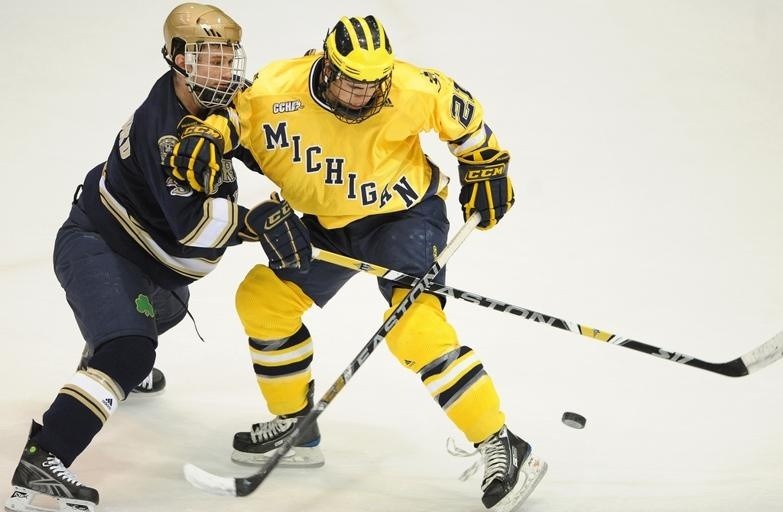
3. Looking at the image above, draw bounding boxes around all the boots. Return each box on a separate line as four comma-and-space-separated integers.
444, 424, 533, 510
233, 378, 323, 455
10, 416, 101, 507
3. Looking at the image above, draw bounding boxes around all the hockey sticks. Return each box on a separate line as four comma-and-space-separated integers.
310, 247, 783, 377
182, 212, 483, 497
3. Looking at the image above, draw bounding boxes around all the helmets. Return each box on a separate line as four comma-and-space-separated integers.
319, 12, 397, 127
160, 1, 251, 110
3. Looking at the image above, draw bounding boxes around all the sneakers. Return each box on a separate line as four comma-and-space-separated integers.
73, 342, 168, 400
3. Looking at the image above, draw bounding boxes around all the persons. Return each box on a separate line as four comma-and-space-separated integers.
162, 14, 533, 510
3, 2, 314, 506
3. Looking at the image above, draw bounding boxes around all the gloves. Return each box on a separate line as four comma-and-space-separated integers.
164, 109, 241, 195
455, 147, 518, 232
235, 188, 316, 280
